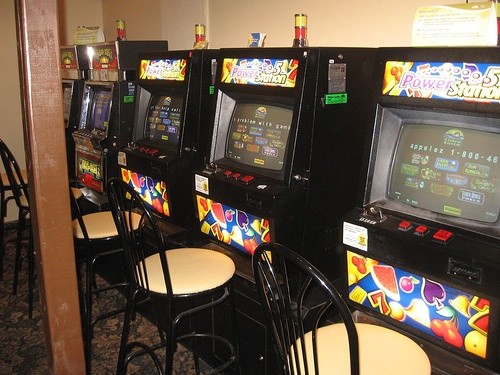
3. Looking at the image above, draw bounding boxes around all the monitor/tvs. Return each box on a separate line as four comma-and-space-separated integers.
225, 97, 295, 181
87, 89, 112, 134
383, 119, 500, 226
142, 91, 185, 147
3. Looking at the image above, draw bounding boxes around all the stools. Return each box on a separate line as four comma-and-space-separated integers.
103, 178, 235, 375
251, 241, 432, 375
69, 180, 146, 375
0, 138, 82, 318
